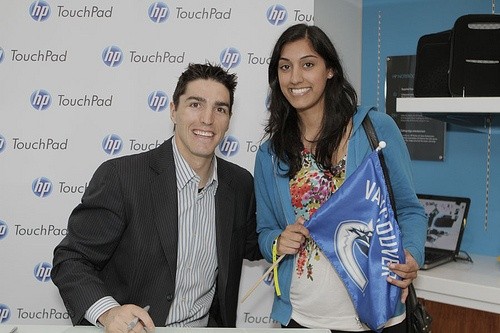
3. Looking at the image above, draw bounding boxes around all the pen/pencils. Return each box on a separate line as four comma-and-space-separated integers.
9, 326, 17, 333
126, 304, 151, 333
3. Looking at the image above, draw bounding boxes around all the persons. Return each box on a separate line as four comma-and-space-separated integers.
49, 62, 265, 333
253, 23, 429, 333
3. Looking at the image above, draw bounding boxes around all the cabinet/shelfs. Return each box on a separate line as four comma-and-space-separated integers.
412, 252, 500, 333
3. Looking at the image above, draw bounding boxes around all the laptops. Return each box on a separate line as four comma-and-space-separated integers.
416, 194, 471, 270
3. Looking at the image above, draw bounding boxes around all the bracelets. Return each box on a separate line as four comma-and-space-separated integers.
272, 236, 282, 297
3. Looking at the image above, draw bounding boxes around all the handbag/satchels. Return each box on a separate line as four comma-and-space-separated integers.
405, 283, 432, 333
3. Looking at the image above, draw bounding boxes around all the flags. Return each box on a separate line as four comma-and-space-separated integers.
303, 148, 405, 330
